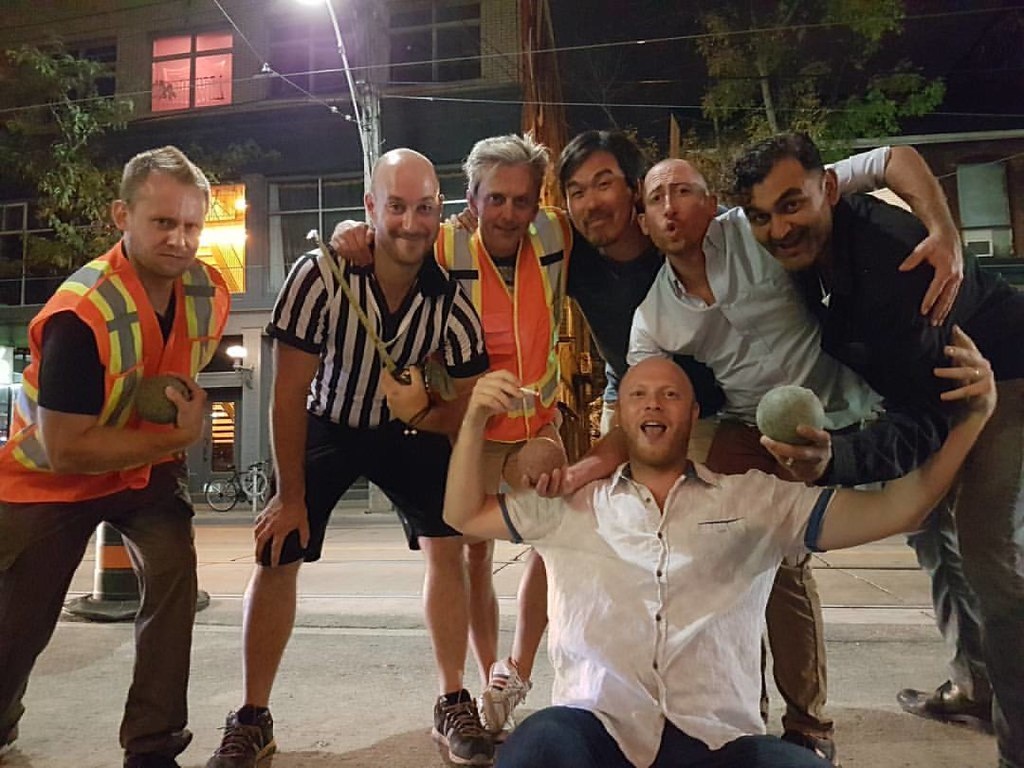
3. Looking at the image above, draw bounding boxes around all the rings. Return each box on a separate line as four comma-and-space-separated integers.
975, 368, 979, 376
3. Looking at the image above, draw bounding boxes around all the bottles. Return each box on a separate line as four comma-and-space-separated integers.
422, 355, 445, 408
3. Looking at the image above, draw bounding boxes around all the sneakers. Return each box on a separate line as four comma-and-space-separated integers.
206, 703, 276, 768
431, 689, 495, 766
492, 714, 518, 743
479, 657, 534, 732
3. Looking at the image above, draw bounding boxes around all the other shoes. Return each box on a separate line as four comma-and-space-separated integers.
122, 755, 179, 768
782, 727, 841, 768
0, 731, 18, 757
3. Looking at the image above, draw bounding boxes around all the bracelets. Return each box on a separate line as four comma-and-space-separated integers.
408, 407, 431, 428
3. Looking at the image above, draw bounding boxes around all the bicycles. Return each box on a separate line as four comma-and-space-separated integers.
204, 458, 273, 513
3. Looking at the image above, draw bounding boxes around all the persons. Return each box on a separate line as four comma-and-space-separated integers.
439, 326, 996, 768
451, 131, 729, 463
735, 131, 1024, 768
521, 146, 964, 768
206, 148, 495, 768
331, 134, 574, 742
0, 146, 231, 768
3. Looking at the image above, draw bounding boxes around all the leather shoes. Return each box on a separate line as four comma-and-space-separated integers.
896, 680, 995, 734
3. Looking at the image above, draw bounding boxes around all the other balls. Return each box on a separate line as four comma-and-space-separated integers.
517, 437, 568, 488
755, 384, 826, 445
133, 375, 190, 425
